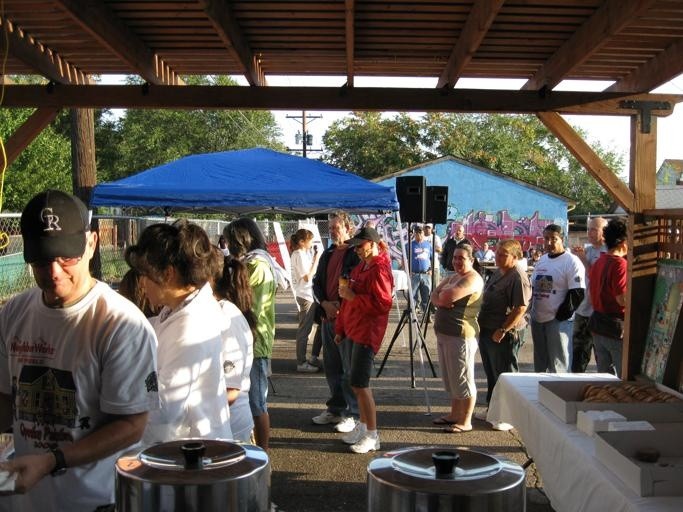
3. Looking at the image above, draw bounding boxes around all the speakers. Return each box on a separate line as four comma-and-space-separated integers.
396, 176, 425, 224
426, 186, 449, 224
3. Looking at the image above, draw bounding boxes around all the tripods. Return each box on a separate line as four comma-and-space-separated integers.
412, 223, 437, 355
375, 222, 437, 388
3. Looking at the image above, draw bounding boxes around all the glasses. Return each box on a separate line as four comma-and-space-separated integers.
33, 256, 79, 266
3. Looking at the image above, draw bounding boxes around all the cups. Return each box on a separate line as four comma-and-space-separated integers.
338, 273, 351, 289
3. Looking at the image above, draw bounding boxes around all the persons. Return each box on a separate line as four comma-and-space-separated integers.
424, 223, 442, 289
116, 222, 233, 446
588, 220, 628, 379
289, 228, 324, 372
523, 247, 542, 267
433, 244, 484, 432
313, 213, 362, 432
475, 242, 496, 262
223, 217, 276, 451
571, 217, 608, 374
478, 238, 533, 431
200, 248, 258, 443
438, 223, 472, 277
0, 190, 158, 512
528, 224, 586, 373
336, 228, 394, 453
402, 226, 435, 323
119, 247, 164, 318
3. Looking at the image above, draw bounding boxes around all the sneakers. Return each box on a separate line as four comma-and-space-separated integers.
341, 424, 366, 443
492, 421, 513, 430
334, 415, 356, 432
350, 434, 381, 453
296, 361, 319, 373
312, 410, 340, 424
476, 409, 488, 420
309, 357, 322, 364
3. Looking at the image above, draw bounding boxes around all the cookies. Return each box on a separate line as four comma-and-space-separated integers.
581, 382, 680, 406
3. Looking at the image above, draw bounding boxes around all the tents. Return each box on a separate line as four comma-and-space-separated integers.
85, 144, 434, 417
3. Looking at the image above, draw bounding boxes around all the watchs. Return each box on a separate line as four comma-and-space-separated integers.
51, 447, 68, 477
498, 326, 507, 335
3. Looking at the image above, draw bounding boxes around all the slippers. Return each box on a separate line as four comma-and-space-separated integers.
432, 417, 456, 424
445, 423, 473, 433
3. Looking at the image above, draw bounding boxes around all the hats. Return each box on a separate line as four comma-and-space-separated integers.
19, 187, 91, 262
344, 228, 379, 246
424, 223, 433, 229
414, 226, 422, 232
